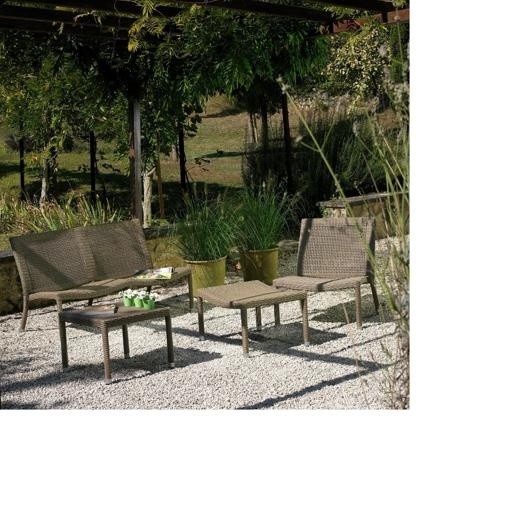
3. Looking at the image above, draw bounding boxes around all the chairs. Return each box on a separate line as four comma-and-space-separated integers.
10, 220, 191, 331
273, 217, 382, 331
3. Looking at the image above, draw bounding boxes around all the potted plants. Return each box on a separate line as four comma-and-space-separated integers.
173, 182, 238, 297
237, 171, 298, 285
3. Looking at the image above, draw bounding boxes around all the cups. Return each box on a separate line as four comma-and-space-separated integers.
168, 266, 176, 273
